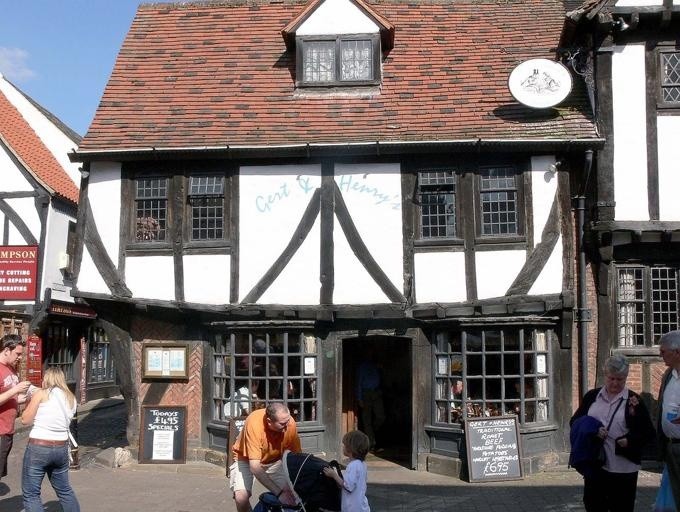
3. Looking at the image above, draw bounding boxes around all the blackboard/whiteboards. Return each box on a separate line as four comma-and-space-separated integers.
464, 415, 525, 483
138, 405, 188, 464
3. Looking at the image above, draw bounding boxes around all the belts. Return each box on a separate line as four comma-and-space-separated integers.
29, 437, 66, 447
664, 438, 680, 443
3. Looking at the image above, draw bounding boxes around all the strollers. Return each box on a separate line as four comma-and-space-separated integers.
253, 449, 343, 512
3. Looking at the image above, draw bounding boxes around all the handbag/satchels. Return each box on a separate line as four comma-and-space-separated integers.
575, 442, 607, 474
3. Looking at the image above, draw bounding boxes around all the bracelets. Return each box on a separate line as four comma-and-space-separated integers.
276, 489, 284, 500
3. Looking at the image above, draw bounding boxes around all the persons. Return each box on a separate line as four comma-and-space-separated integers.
224, 339, 312, 420
568, 352, 656, 511
446, 380, 463, 413
20, 366, 80, 512
513, 380, 534, 422
653, 329, 680, 512
355, 345, 386, 454
229, 402, 303, 512
0, 334, 31, 482
321, 429, 372, 512
